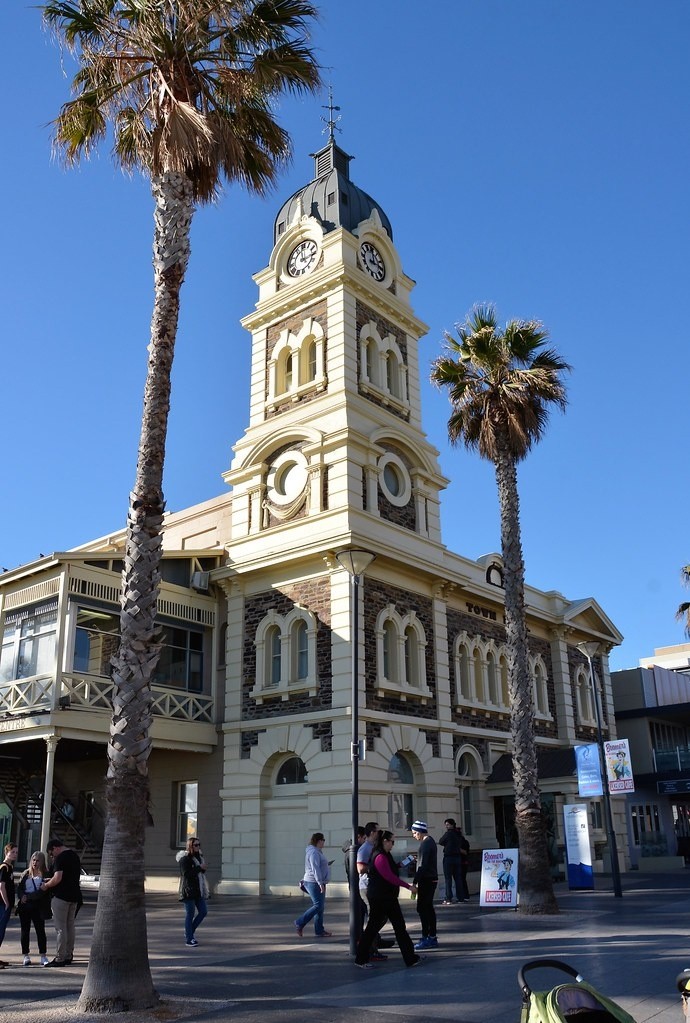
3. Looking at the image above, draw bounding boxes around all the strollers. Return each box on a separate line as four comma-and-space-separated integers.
517, 959, 636, 1023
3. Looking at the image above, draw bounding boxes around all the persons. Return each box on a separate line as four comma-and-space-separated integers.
38, 839, 81, 968
293, 833, 332, 938
51, 798, 76, 825
342, 826, 366, 944
411, 820, 440, 949
356, 822, 388, 961
453, 827, 470, 903
353, 830, 428, 970
0, 842, 18, 969
439, 818, 466, 906
17, 850, 53, 965
176, 838, 210, 948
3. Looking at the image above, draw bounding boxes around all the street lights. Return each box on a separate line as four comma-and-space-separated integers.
334, 548, 377, 957
575, 639, 624, 900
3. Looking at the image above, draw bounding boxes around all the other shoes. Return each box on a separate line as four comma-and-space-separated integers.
316, 930, 332, 937
442, 900, 452, 905
23, 956, 31, 965
458, 900, 464, 904
409, 954, 428, 967
370, 952, 388, 961
41, 957, 50, 965
293, 920, 303, 936
0, 960, 8, 968
464, 898, 469, 901
184, 933, 199, 947
45, 957, 72, 967
354, 960, 376, 969
378, 939, 395, 948
413, 936, 439, 949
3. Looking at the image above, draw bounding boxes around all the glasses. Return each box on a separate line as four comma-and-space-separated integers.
192, 844, 200, 847
319, 838, 326, 841
385, 838, 394, 845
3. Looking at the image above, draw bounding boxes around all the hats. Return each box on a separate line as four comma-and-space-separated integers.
412, 820, 428, 834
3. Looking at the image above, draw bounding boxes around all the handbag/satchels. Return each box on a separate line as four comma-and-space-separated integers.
299, 879, 309, 894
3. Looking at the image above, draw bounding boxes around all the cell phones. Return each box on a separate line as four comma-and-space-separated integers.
402, 855, 414, 865
328, 860, 335, 865
